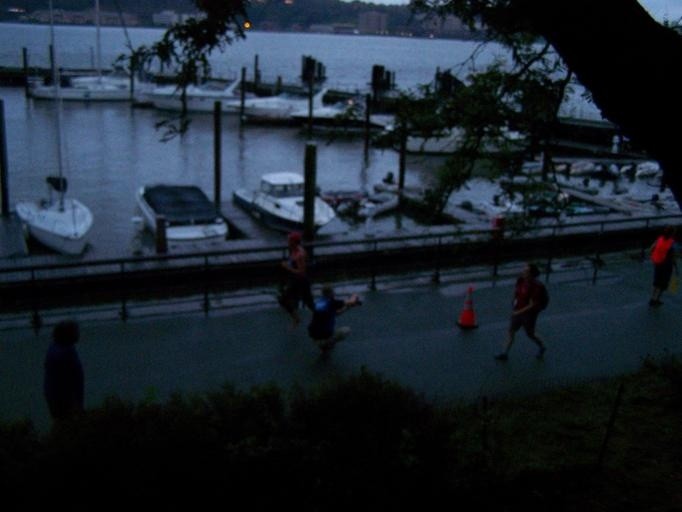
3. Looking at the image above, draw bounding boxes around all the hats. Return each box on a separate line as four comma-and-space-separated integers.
288, 231, 302, 240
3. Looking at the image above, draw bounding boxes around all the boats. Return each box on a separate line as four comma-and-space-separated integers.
131, 183, 228, 247
15, 176, 94, 256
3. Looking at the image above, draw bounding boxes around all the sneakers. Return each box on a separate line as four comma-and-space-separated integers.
648, 298, 663, 309
493, 344, 547, 359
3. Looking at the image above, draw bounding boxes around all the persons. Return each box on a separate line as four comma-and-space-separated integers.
42, 315, 85, 422
643, 222, 682, 309
308, 282, 361, 368
279, 232, 316, 324
491, 263, 545, 362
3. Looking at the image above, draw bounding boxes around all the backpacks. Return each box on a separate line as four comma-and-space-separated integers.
526, 279, 549, 314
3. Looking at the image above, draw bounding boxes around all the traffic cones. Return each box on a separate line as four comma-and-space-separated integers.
457, 287, 479, 328
